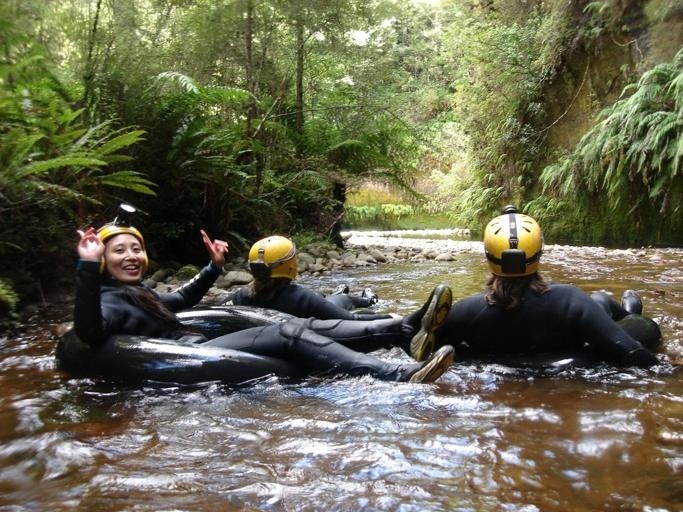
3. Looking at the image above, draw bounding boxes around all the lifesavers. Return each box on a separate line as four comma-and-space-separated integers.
56, 306, 299, 389
403, 315, 663, 370
225, 307, 404, 355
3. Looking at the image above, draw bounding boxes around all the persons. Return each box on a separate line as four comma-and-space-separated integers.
73, 202, 454, 385
218, 233, 404, 321
432, 205, 682, 377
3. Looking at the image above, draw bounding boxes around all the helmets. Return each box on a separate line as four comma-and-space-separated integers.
483, 204, 544, 278
248, 236, 298, 282
95, 204, 150, 277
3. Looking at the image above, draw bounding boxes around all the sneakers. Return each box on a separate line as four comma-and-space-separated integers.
361, 287, 378, 304
621, 289, 642, 314
398, 344, 456, 383
335, 284, 349, 295
400, 284, 453, 362
